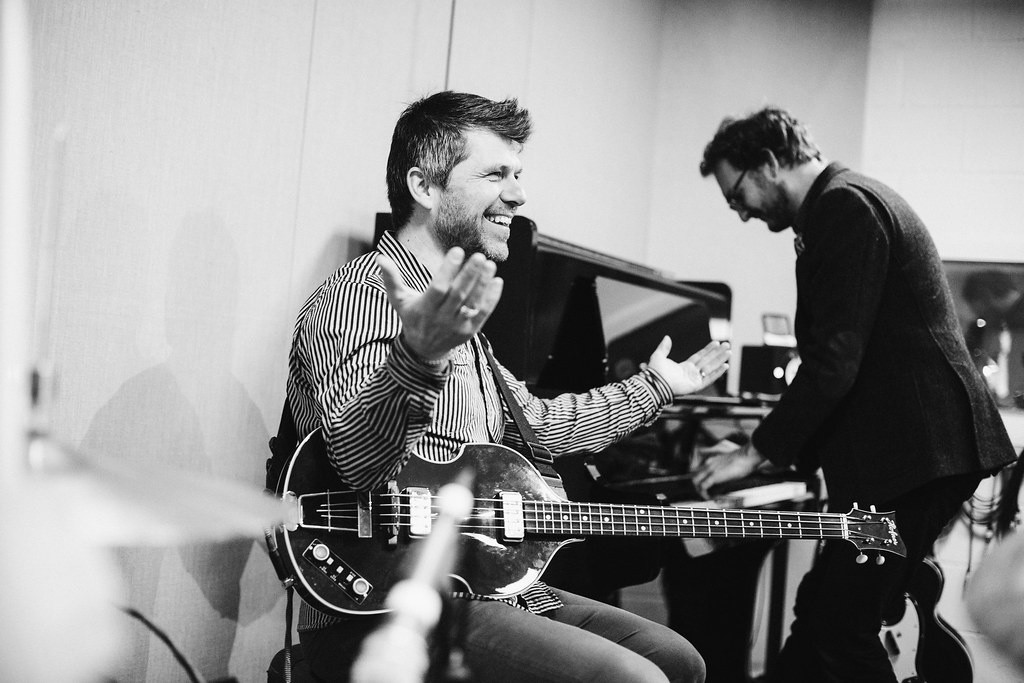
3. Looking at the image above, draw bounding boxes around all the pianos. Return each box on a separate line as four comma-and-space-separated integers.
369, 205, 818, 683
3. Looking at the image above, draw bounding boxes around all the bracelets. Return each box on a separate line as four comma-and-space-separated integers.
421, 355, 449, 366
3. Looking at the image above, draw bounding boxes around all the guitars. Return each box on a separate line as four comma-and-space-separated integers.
264, 424, 910, 618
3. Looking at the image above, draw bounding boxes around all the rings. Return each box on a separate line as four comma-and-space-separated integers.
461, 305, 478, 318
700, 370, 705, 377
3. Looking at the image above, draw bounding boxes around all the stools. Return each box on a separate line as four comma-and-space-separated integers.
269, 643, 325, 683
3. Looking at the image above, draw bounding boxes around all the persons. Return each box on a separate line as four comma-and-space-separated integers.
964, 265, 1024, 404
288, 93, 732, 683
690, 107, 1017, 683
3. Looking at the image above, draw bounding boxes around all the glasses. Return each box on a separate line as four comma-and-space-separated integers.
727, 155, 756, 211
